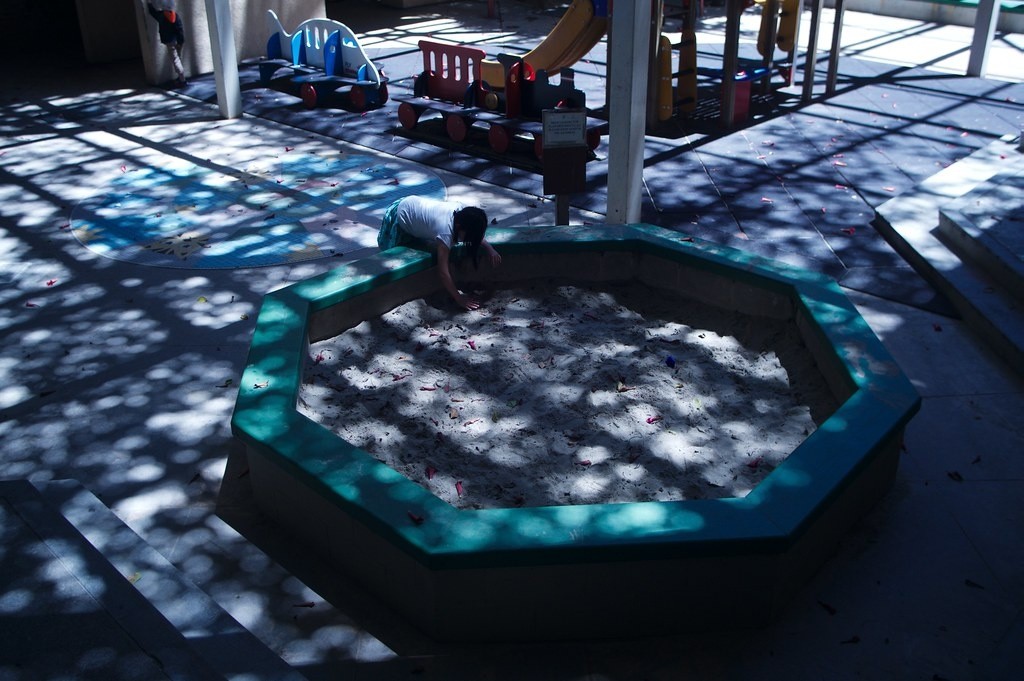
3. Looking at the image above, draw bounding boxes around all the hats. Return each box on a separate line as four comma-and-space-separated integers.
163, 0, 175, 11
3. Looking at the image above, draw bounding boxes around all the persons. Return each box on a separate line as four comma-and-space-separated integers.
146, 0, 185, 84
376, 195, 502, 311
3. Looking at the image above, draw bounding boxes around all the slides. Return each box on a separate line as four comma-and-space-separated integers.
473, 0, 610, 90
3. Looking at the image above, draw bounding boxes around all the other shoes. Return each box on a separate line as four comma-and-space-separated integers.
169, 79, 187, 87
